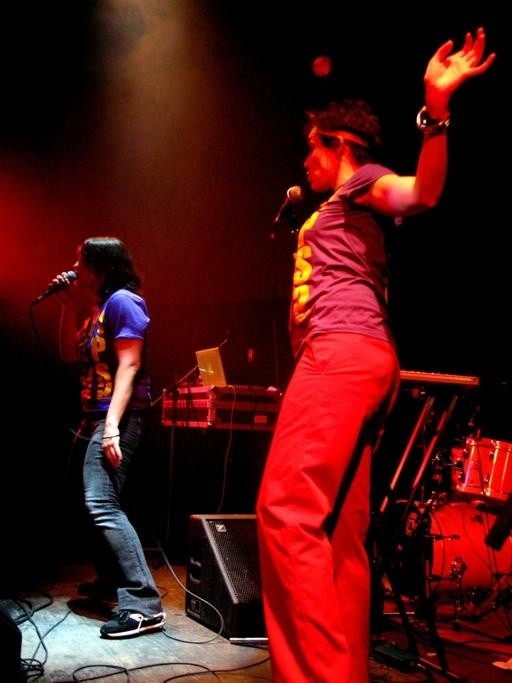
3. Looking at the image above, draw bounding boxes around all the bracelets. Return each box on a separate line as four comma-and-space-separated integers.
101, 431, 123, 440
415, 105, 453, 135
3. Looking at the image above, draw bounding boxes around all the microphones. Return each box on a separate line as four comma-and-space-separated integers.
37, 270, 77, 304
272, 184, 305, 226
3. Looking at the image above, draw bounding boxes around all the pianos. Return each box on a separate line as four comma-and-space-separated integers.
398, 366, 481, 389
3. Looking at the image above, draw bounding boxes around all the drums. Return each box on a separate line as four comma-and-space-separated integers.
453, 434, 512, 507
378, 500, 512, 600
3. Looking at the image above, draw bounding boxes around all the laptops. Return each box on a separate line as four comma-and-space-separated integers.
194, 344, 233, 387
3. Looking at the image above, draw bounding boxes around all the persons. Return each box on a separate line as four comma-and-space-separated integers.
255, 28, 499, 683
52, 238, 166, 638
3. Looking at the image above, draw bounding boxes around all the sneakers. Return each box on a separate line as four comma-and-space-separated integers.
78, 581, 121, 601
100, 610, 166, 637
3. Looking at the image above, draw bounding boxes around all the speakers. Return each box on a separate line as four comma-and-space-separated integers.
185, 514, 267, 640
